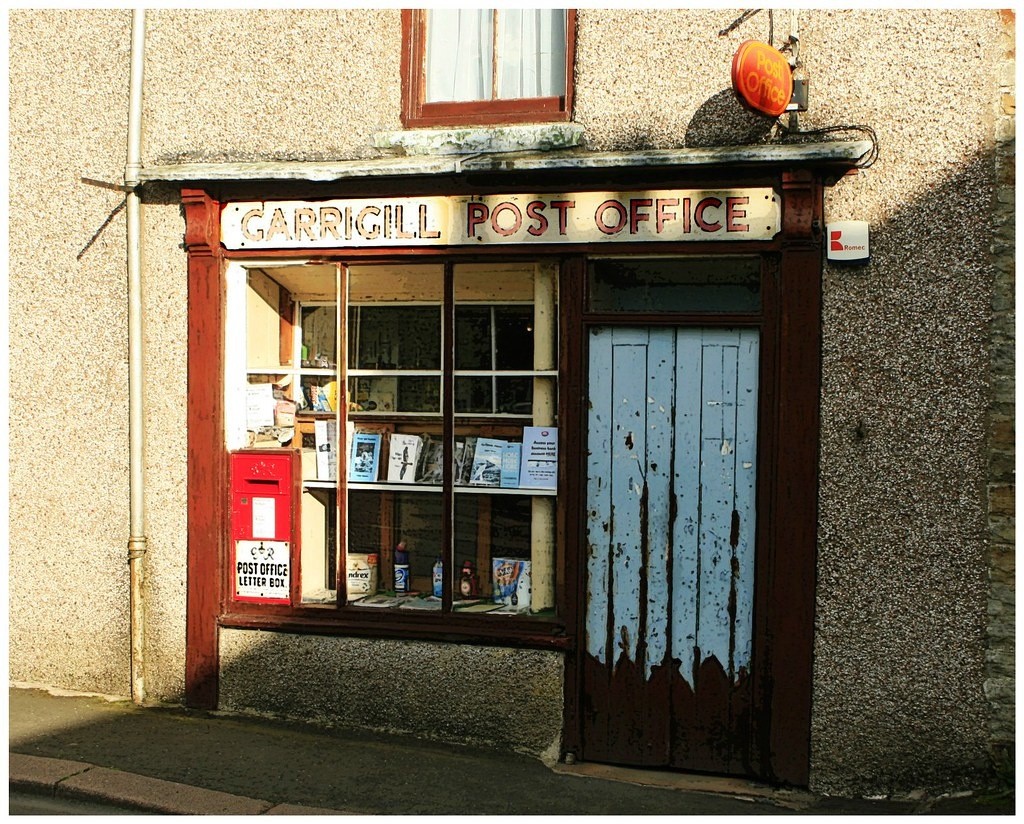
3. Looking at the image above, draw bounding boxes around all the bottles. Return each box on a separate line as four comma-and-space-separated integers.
394, 546, 410, 592
461, 561, 477, 599
432, 557, 443, 596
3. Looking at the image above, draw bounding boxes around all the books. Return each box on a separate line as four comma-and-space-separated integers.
313, 416, 557, 492
492, 557, 532, 608
